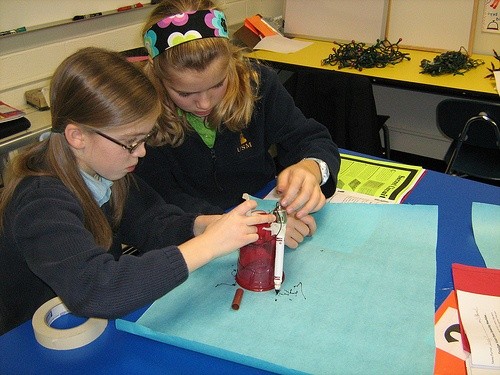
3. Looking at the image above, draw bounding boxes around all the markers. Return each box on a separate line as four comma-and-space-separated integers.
0, 26, 27, 36
273, 221, 287, 297
73, 12, 102, 20
118, 2, 143, 11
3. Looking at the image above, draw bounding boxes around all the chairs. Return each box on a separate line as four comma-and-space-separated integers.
435, 98, 500, 188
277, 69, 390, 159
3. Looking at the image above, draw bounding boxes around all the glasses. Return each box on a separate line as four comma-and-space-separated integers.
89, 124, 158, 153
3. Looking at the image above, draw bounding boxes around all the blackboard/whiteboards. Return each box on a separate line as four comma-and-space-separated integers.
284, 0, 387, 45
384, 0, 476, 55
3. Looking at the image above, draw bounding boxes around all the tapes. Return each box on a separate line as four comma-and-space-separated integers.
32, 296, 108, 351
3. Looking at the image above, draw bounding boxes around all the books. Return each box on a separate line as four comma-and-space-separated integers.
262, 153, 427, 205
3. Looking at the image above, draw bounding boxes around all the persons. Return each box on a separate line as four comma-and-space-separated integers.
134, 0, 341, 249
0, 47, 276, 337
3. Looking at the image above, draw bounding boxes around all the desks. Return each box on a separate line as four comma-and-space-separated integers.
238, 32, 500, 103
1, 148, 500, 374
